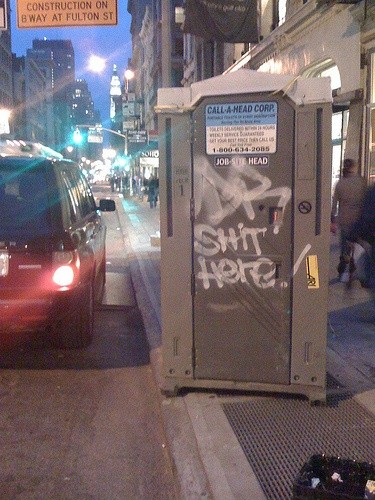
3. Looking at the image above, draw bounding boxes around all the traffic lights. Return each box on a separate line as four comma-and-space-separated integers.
73, 131, 81, 143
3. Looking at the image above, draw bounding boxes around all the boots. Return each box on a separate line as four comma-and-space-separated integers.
349, 258, 356, 273
338, 256, 347, 274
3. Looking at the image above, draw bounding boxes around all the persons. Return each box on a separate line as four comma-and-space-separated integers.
109, 170, 159, 209
330, 160, 370, 284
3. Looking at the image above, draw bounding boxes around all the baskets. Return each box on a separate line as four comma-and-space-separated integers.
295, 453, 375, 500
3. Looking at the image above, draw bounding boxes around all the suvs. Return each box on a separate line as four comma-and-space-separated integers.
1, 156, 115, 351
